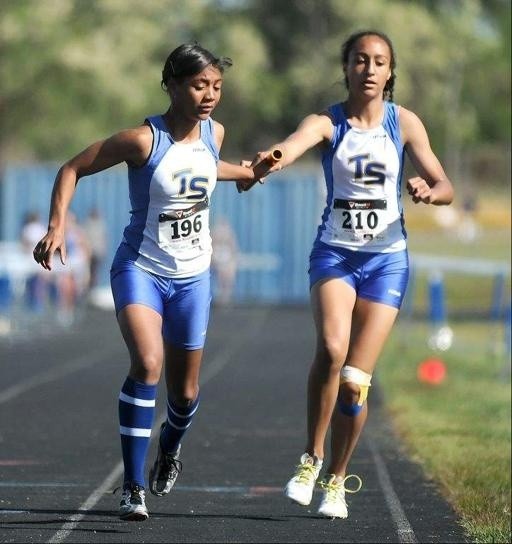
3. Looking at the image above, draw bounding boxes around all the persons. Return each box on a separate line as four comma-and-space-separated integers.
0, 201, 108, 336
212, 211, 241, 312
33, 40, 280, 520
237, 30, 455, 524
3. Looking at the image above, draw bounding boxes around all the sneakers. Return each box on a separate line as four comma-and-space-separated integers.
284, 453, 323, 505
119, 483, 149, 521
149, 422, 181, 496
317, 485, 348, 518
